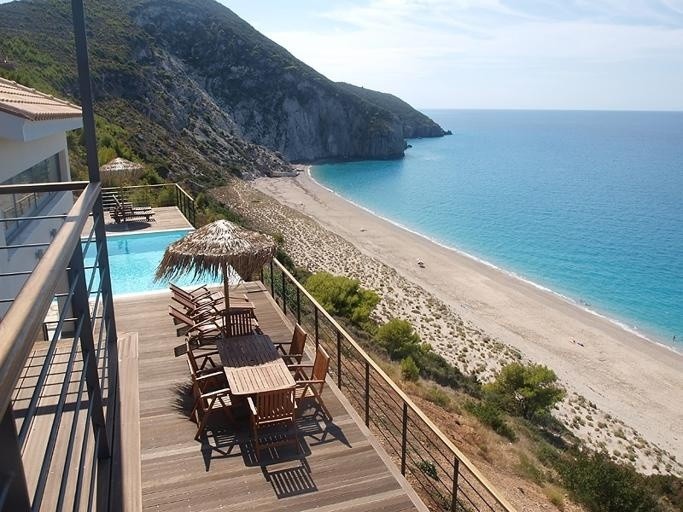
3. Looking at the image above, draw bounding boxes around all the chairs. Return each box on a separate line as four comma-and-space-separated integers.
165, 279, 332, 460
107, 194, 157, 226
